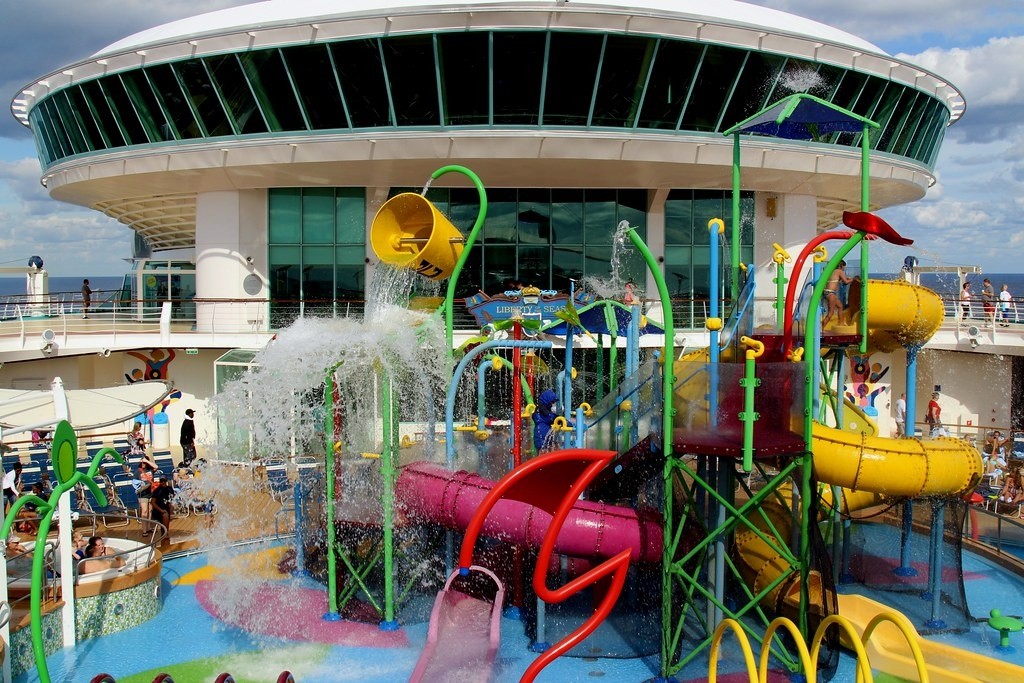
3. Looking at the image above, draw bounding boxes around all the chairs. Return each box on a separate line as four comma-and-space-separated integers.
253, 453, 324, 506
4, 437, 218, 533
974, 430, 1024, 519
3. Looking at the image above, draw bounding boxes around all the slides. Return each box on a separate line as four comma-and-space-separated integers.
267, 273, 1024, 682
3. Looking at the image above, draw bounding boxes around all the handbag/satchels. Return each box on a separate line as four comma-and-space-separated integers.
924, 414, 929, 423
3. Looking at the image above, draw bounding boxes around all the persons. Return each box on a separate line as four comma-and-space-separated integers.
147, 475, 175, 538
172, 458, 213, 515
818, 260, 853, 329
981, 279, 994, 329
1002, 466, 1019, 496
133, 456, 158, 537
997, 284, 1012, 327
31, 430, 52, 446
985, 456, 994, 475
156, 278, 180, 320
2, 440, 133, 586
927, 392, 942, 429
82, 279, 93, 319
180, 408, 196, 466
895, 392, 906, 437
532, 389, 573, 456
963, 435, 970, 444
960, 282, 972, 327
499, 277, 634, 308
984, 430, 1008, 458
126, 422, 151, 455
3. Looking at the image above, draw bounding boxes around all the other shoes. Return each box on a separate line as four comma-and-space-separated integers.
998, 323, 1010, 327
82, 315, 89, 319
981, 324, 992, 328
960, 323, 970, 327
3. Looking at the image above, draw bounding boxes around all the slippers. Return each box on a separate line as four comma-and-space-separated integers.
142, 529, 158, 537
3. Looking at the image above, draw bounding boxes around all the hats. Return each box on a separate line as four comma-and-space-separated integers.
185, 409, 196, 415
